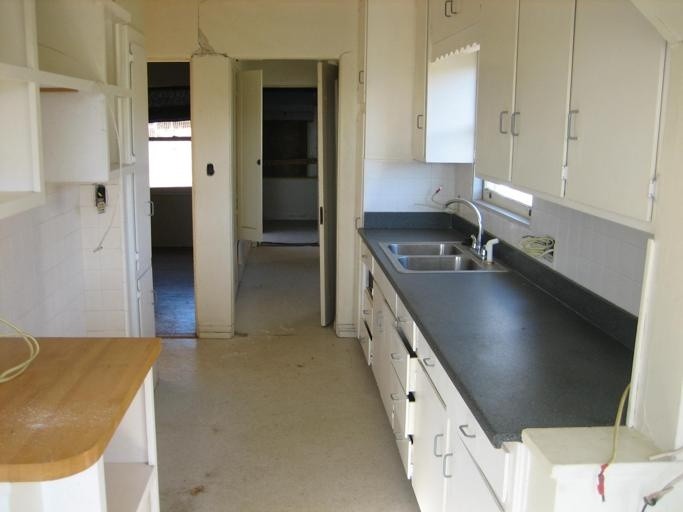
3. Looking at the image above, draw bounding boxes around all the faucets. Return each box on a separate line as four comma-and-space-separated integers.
440, 197, 484, 254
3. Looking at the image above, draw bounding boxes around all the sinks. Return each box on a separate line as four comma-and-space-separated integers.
386, 255, 509, 274
377, 239, 469, 256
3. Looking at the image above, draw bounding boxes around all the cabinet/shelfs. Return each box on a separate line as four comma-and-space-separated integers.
371, 257, 414, 476
360, 240, 375, 365
0, 1, 111, 220
112, 24, 158, 339
565, 0, 666, 236
413, 0, 474, 164
410, 329, 514, 512
474, 0, 576, 205
351, 0, 413, 336
428, 0, 481, 63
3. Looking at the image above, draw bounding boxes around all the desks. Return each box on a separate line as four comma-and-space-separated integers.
0, 335, 158, 511
